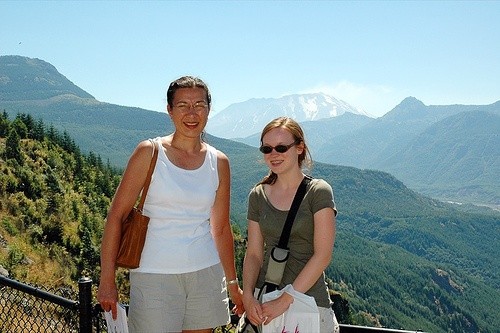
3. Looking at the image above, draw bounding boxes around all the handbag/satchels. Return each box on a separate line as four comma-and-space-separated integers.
235, 282, 279, 333
116, 142, 159, 269
262, 285, 320, 333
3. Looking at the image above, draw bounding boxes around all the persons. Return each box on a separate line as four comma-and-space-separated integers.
97, 76, 245, 333
241, 117, 339, 333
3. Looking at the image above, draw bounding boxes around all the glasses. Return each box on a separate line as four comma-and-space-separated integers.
173, 103, 206, 113
260, 142, 296, 154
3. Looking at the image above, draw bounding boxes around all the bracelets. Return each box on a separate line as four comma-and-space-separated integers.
228, 278, 238, 285
